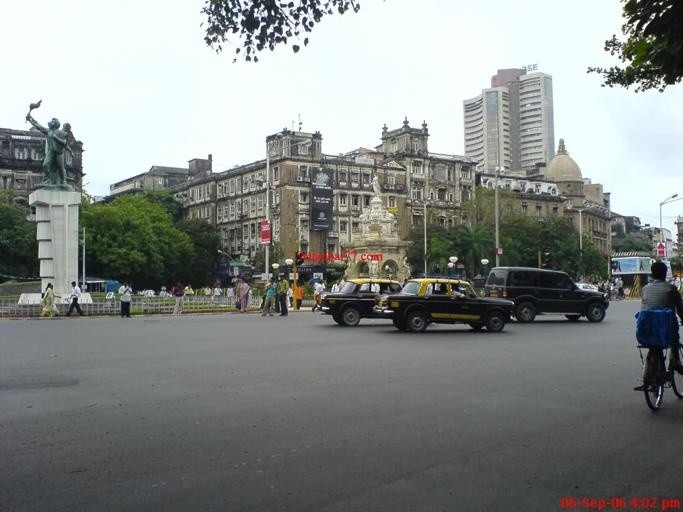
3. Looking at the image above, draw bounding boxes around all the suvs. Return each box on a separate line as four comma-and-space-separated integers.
575, 283, 597, 291
482, 265, 608, 323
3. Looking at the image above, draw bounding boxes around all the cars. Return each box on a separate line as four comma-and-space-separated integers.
312, 279, 403, 328
376, 278, 515, 334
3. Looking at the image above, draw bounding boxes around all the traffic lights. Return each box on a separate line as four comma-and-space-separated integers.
294, 251, 304, 266
543, 252, 552, 269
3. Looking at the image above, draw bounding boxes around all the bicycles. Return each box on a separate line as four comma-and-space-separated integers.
632, 339, 682, 413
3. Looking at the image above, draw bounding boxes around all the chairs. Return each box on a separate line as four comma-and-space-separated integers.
440, 284, 448, 294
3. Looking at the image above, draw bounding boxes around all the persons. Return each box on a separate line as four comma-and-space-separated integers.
26, 114, 69, 185
598, 276, 625, 301
158, 274, 348, 316
64, 282, 85, 317
41, 284, 62, 317
118, 280, 133, 318
452, 284, 466, 299
641, 262, 683, 376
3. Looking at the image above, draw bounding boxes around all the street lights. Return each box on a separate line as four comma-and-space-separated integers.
446, 255, 489, 278
284, 257, 292, 287
414, 195, 435, 278
271, 263, 280, 312
217, 249, 237, 279
262, 138, 311, 282
492, 167, 506, 268
568, 205, 591, 258
658, 192, 682, 243
79, 226, 87, 293
